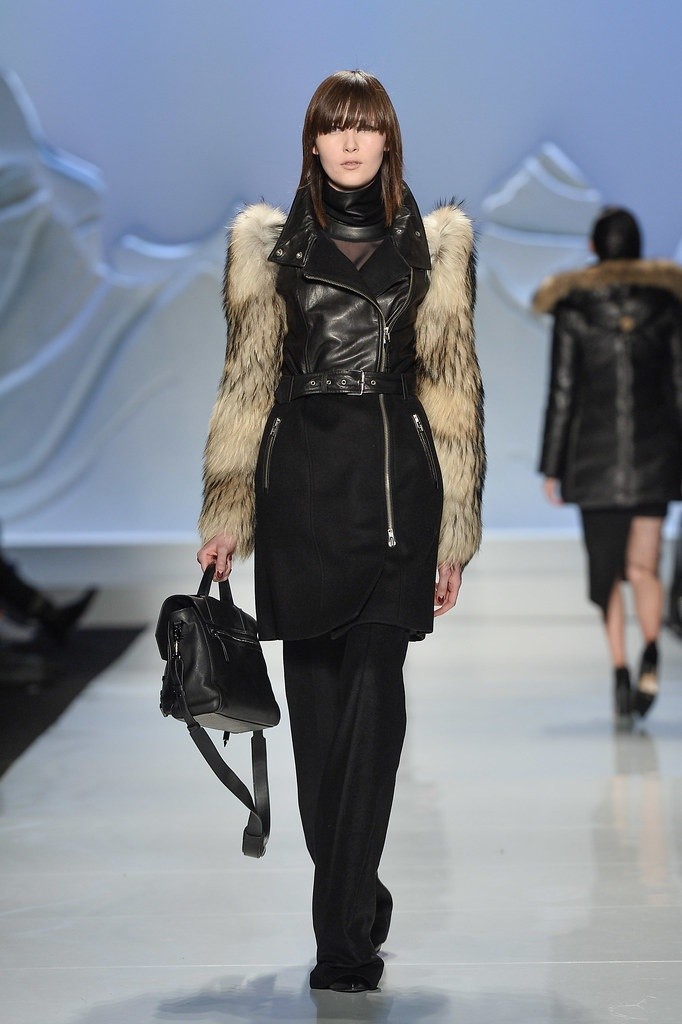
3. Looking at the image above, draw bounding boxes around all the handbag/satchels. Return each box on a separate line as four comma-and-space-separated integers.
154, 562, 280, 734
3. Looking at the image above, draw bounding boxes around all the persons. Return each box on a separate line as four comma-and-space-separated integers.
531, 205, 682, 730
198, 68, 486, 993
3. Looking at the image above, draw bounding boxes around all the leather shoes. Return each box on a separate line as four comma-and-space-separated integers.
329, 976, 368, 994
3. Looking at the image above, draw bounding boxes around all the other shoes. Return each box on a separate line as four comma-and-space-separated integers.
634, 641, 659, 714
615, 668, 632, 714
37, 587, 99, 652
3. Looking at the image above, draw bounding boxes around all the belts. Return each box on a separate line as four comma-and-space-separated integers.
275, 370, 415, 400
